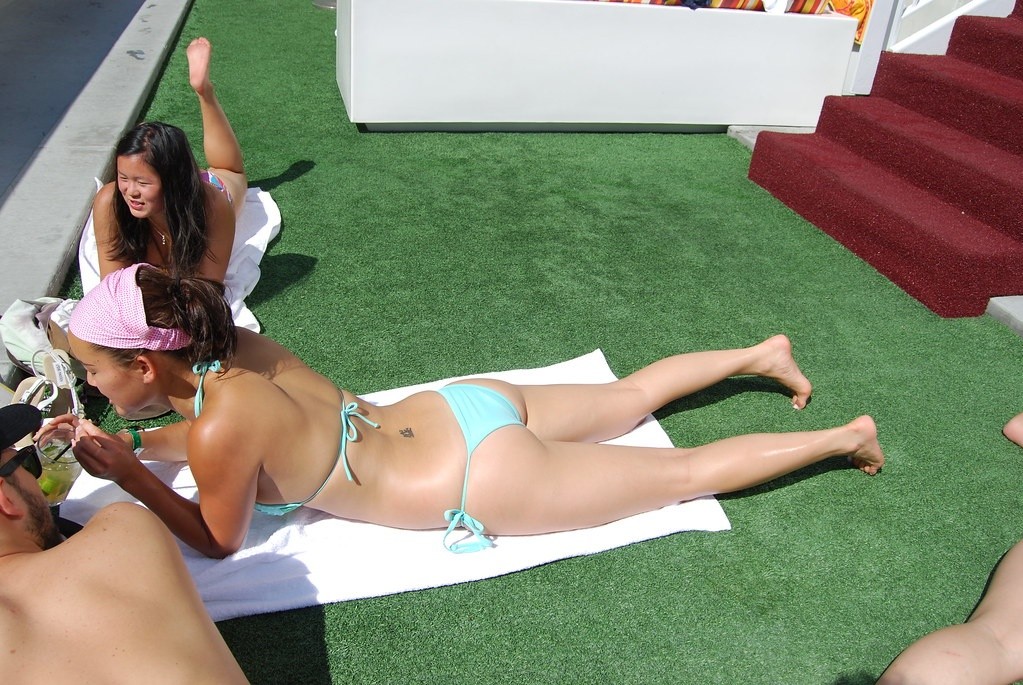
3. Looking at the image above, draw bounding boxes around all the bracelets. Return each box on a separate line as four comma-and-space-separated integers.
127, 429, 142, 453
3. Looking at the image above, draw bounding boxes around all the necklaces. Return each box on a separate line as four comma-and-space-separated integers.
151, 223, 166, 245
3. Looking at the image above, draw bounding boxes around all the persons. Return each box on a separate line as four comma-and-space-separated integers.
873, 407, 1023, 685
90, 37, 248, 284
0, 403, 251, 685
32, 263, 882, 559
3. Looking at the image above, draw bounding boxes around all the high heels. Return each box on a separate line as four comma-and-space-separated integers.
11, 349, 84, 447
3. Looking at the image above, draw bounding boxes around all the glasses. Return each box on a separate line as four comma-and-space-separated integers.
0, 445, 42, 480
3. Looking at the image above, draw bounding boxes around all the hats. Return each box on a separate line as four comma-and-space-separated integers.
0, 403, 42, 450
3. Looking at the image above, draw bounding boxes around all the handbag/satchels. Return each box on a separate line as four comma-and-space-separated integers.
0, 294, 89, 383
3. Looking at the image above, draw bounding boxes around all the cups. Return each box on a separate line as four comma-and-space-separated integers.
32, 428, 86, 508
